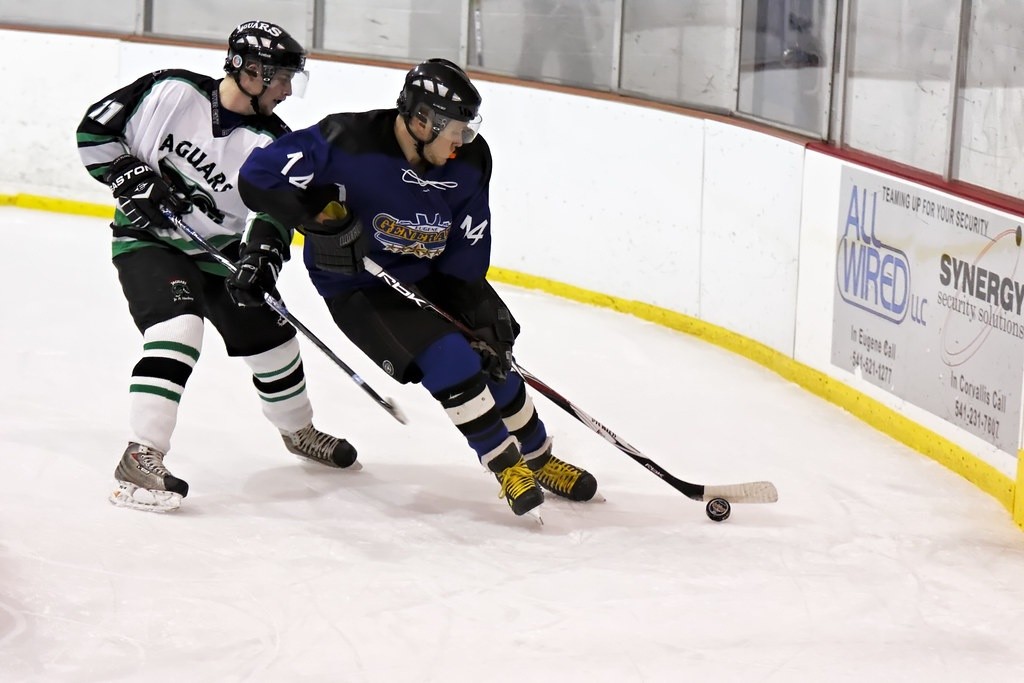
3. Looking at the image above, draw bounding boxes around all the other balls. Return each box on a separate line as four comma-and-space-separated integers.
706, 498, 731, 521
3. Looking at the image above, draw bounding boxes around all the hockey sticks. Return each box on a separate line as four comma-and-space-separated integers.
361, 254, 778, 504
157, 203, 409, 425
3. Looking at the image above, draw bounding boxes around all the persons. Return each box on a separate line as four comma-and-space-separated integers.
76, 21, 363, 513
237, 57, 607, 527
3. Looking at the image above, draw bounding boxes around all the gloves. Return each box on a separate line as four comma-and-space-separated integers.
226, 212, 292, 324
104, 154, 183, 230
295, 207, 365, 275
470, 340, 511, 384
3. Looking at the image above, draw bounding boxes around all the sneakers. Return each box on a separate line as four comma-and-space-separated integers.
109, 442, 188, 513
523, 435, 606, 503
279, 421, 362, 471
480, 436, 544, 526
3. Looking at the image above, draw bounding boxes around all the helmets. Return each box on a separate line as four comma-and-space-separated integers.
398, 58, 482, 121
224, 22, 309, 75
